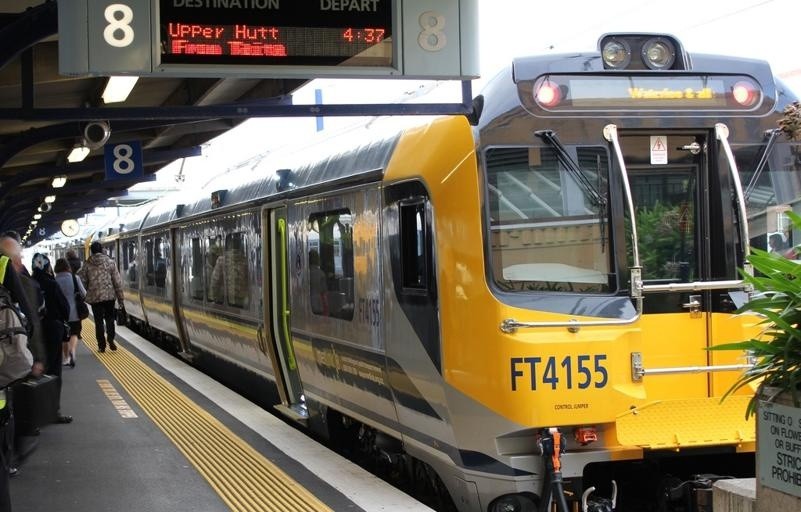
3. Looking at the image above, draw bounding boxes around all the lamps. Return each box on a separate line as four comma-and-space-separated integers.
21, 75, 139, 243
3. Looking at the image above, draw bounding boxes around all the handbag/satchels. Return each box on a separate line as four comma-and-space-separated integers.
116, 308, 128, 325
74, 293, 89, 319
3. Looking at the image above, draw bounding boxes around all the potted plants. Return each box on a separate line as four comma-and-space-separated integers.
703, 210, 801, 512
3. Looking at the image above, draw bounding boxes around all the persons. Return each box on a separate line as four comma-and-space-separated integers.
0, 230, 88, 512
309, 249, 330, 316
207, 238, 250, 308
79, 241, 124, 353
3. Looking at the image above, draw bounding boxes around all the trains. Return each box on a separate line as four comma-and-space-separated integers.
33, 32, 801, 512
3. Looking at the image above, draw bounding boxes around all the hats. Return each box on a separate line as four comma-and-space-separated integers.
32, 253, 49, 272
54, 258, 70, 270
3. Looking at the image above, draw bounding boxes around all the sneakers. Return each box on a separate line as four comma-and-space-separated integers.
98, 338, 117, 353
62, 350, 77, 367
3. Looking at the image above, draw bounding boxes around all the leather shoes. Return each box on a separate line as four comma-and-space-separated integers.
49, 414, 72, 424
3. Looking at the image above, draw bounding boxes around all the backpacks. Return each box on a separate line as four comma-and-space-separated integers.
0, 282, 34, 390
